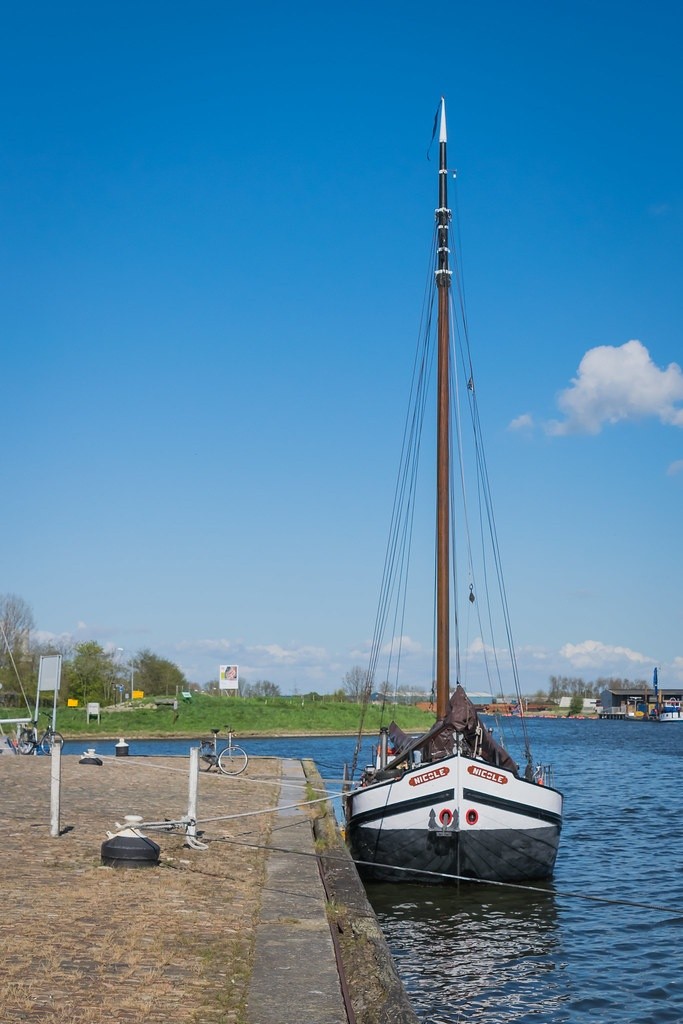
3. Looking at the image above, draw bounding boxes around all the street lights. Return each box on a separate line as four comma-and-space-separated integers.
117, 648, 134, 700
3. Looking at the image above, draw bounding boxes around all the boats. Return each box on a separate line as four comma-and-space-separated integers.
661, 702, 683, 721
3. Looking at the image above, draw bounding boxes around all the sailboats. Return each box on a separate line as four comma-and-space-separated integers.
346, 100, 563, 881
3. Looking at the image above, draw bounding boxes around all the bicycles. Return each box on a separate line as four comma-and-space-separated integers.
18, 711, 65, 755
196, 725, 248, 776
